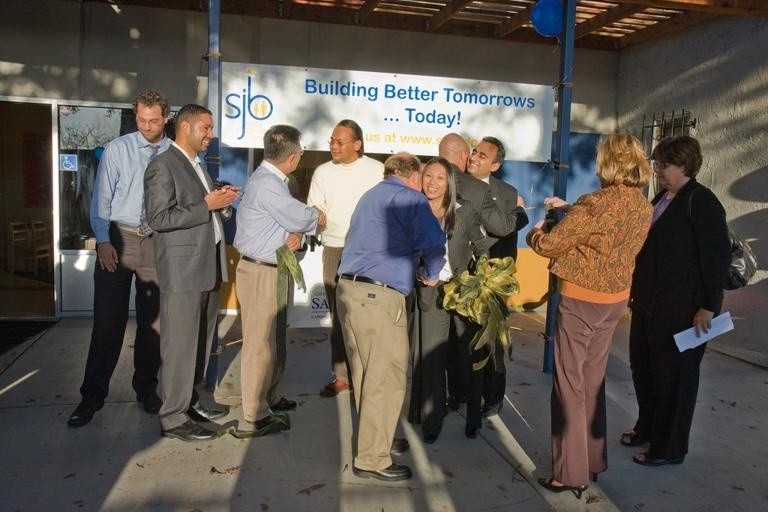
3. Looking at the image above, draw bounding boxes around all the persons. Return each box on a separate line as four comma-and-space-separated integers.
527, 130, 652, 498
145, 102, 243, 441
620, 135, 734, 467
336, 131, 532, 482
68, 90, 176, 426
229, 124, 327, 433
308, 123, 387, 400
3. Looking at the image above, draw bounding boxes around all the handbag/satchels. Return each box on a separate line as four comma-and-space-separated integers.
687, 186, 757, 291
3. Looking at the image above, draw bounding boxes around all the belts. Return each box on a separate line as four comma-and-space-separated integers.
119, 224, 145, 238
243, 256, 277, 267
342, 273, 388, 288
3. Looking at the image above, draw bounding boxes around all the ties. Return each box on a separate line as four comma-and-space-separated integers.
139, 145, 161, 238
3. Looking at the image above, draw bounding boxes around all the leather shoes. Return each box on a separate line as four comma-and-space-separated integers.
422, 397, 502, 444
620, 430, 648, 447
391, 438, 409, 453
353, 465, 411, 482
632, 450, 685, 467
68, 399, 104, 427
137, 391, 162, 413
160, 398, 296, 443
319, 378, 347, 397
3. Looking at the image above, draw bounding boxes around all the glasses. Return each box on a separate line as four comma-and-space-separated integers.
652, 162, 675, 169
299, 149, 304, 156
328, 137, 344, 146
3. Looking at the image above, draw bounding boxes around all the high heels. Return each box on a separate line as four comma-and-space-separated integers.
538, 477, 588, 499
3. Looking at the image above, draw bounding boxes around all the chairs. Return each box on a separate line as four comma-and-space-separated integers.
7, 219, 53, 279
